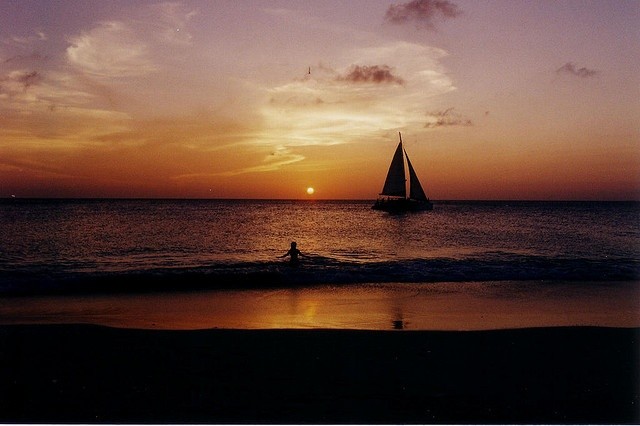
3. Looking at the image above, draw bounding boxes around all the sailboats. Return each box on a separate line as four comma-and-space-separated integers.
371, 131, 433, 215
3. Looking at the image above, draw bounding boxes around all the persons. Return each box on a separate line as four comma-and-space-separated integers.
275, 242, 310, 262
